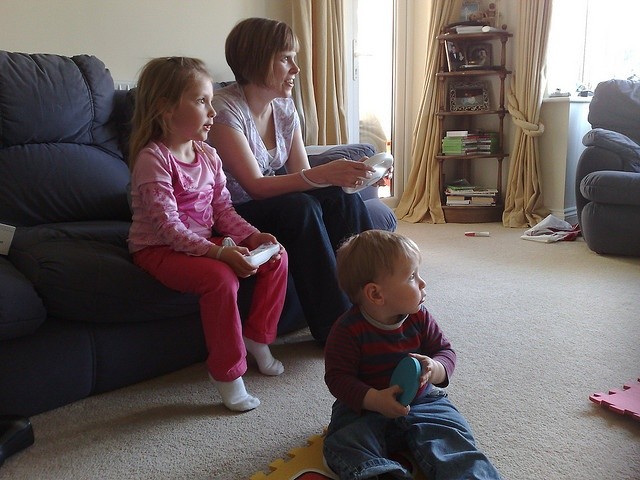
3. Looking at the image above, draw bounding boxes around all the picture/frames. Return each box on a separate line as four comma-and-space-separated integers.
460, 2, 479, 21
469, 43, 492, 68
444, 40, 468, 72
450, 80, 489, 112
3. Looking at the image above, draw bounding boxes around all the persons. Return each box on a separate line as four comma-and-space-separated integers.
457, 51, 465, 62
449, 45, 459, 62
203, 16, 394, 350
127, 56, 290, 412
322, 230, 501, 480
461, 92, 478, 107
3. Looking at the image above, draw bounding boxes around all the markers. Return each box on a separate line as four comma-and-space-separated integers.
465, 232, 490, 237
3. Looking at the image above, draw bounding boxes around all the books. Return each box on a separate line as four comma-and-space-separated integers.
442, 130, 500, 157
445, 179, 498, 207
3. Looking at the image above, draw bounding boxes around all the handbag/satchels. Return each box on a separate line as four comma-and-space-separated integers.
519, 213, 581, 245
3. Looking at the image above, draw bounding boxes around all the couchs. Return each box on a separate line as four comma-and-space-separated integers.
575, 79, 640, 256
1, 50, 397, 424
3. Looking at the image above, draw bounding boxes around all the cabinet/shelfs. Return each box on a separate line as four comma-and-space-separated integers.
434, 23, 513, 224
